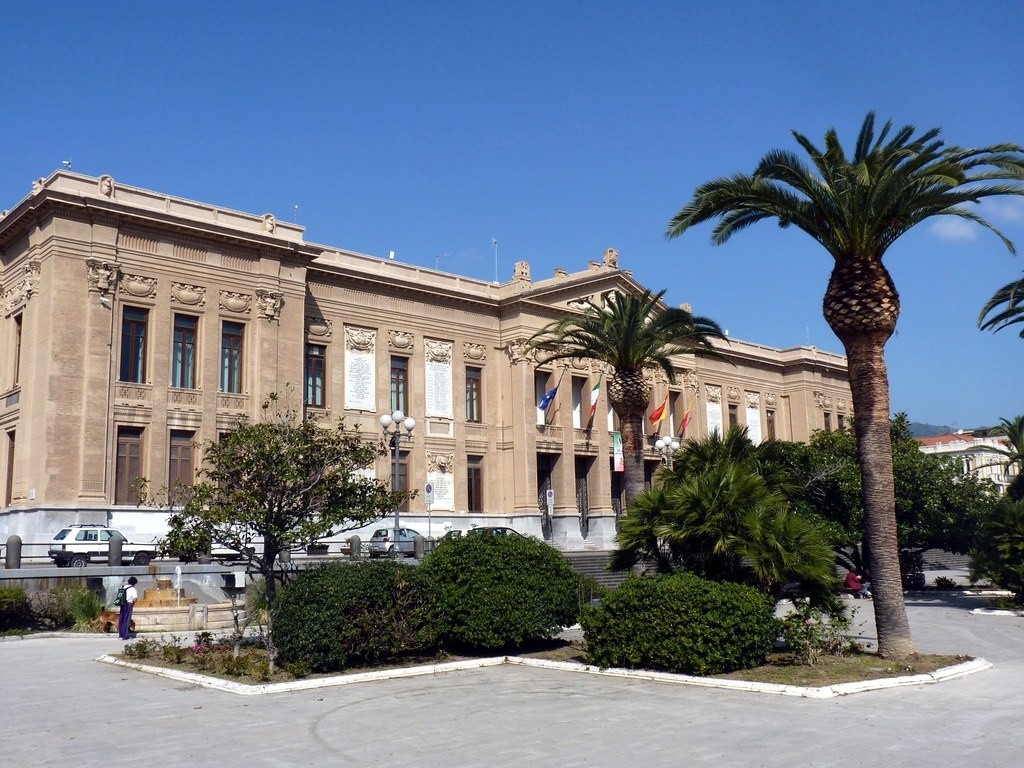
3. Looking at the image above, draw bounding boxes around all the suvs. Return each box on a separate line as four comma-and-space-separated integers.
48, 523, 159, 565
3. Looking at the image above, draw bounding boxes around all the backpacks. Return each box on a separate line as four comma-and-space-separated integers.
112, 585, 134, 606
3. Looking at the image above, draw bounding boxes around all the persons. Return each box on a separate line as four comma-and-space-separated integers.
114, 577, 137, 642
846, 565, 871, 600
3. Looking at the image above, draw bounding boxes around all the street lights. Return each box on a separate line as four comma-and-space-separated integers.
380, 410, 418, 560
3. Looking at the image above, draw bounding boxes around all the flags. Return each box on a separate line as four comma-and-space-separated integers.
682, 394, 695, 434
589, 372, 601, 420
538, 387, 556, 411
649, 391, 667, 426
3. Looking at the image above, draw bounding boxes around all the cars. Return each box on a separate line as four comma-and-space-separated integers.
435, 528, 476, 547
472, 527, 529, 540
368, 528, 425, 558
209, 536, 251, 561
